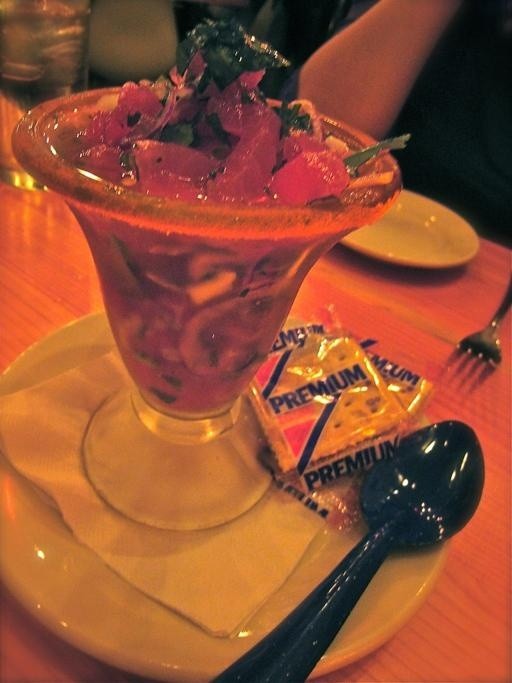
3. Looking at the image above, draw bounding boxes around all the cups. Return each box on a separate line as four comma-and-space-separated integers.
13, 85, 403, 531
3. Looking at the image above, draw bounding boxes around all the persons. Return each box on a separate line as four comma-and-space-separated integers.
262, 0, 510, 252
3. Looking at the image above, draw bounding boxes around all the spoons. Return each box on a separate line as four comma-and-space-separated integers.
213, 419, 486, 677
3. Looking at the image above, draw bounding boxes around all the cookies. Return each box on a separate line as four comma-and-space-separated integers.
249, 337, 404, 473
257, 350, 432, 516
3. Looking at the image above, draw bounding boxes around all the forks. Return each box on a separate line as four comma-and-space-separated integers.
431, 274, 512, 397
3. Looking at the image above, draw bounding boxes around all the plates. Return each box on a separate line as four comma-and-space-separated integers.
2, 306, 451, 677
340, 187, 482, 274
